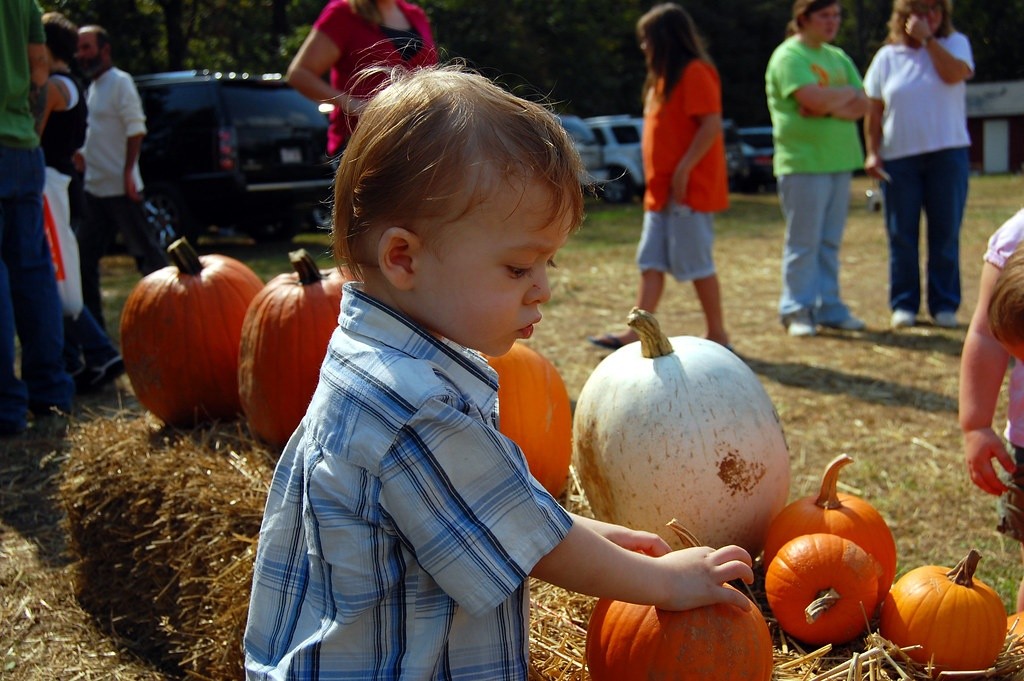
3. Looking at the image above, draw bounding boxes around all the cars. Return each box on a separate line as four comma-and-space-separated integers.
720, 121, 773, 195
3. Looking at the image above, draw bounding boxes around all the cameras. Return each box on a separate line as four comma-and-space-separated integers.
868, 191, 881, 212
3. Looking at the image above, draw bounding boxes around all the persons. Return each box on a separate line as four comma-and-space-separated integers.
245, 58, 754, 681
286, 1, 438, 170
863, 1, 975, 330
765, 0, 871, 337
588, 5, 730, 350
0, 0, 170, 434
958, 208, 1024, 541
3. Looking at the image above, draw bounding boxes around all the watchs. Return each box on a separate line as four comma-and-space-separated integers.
921, 34, 932, 49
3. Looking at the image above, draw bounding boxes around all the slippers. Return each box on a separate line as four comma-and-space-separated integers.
587, 334, 625, 351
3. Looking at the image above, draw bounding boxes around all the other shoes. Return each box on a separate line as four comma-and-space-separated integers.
30, 405, 71, 437
891, 307, 958, 328
784, 308, 865, 337
61, 345, 125, 387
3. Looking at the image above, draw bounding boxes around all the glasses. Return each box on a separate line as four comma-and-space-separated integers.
911, 2, 943, 13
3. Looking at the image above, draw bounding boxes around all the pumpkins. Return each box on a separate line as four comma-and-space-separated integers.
765, 533, 879, 647
584, 518, 774, 681
571, 307, 792, 562
119, 237, 264, 424
239, 250, 351, 450
764, 452, 897, 608
474, 340, 571, 500
877, 548, 1024, 681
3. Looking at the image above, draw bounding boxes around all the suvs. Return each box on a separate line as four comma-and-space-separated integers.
559, 112, 609, 187
584, 116, 645, 204
131, 66, 335, 244
